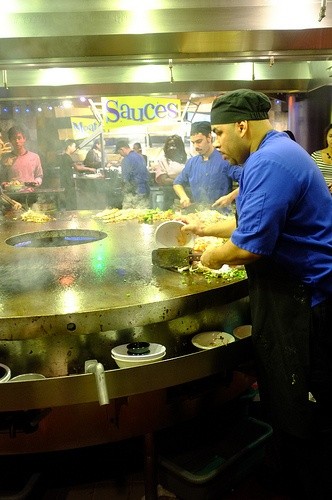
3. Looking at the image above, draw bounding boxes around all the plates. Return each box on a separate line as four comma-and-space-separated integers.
233, 325, 252, 339
155, 221, 191, 246
191, 331, 235, 349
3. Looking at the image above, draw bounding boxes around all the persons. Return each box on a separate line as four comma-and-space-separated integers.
174, 120, 243, 212
113, 141, 150, 200
0, 128, 22, 210
311, 122, 332, 196
173, 90, 332, 444
1, 126, 43, 207
59, 139, 96, 209
133, 143, 148, 166
84, 138, 112, 168
155, 135, 195, 185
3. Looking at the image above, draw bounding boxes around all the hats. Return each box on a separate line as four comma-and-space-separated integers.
191, 121, 211, 133
63, 139, 73, 149
133, 143, 141, 149
113, 141, 129, 154
210, 88, 271, 125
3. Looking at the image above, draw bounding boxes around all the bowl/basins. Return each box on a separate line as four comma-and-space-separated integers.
111, 342, 166, 368
8, 185, 24, 190
